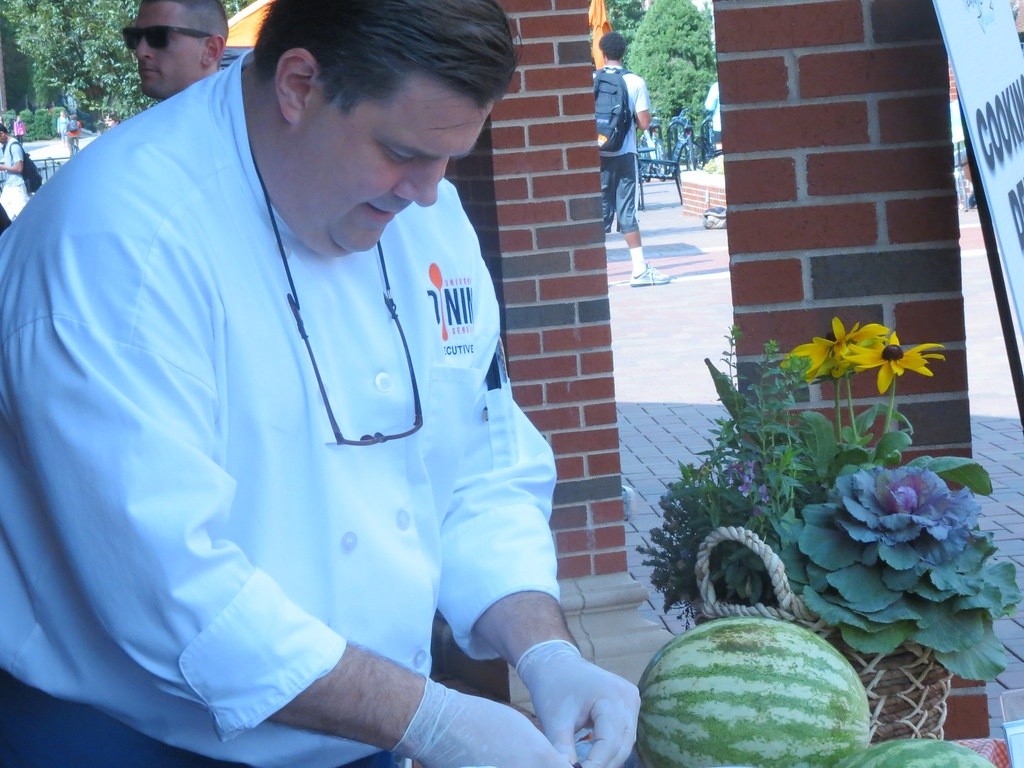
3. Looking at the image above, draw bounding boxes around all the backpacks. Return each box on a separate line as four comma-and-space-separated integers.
593, 66, 633, 152
68, 119, 78, 132
2, 141, 42, 193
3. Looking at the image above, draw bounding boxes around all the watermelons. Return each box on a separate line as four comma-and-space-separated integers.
634, 616, 873, 768
830, 738, 1000, 768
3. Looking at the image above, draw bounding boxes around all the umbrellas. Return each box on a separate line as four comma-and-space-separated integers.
589, 0, 613, 70
0, 0, 639, 768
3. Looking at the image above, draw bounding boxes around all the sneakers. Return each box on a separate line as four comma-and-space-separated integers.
630, 261, 671, 285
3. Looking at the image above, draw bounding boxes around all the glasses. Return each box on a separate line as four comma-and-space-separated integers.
286, 291, 423, 447
122, 23, 211, 50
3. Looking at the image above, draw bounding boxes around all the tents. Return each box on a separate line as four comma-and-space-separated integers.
220, 0, 275, 66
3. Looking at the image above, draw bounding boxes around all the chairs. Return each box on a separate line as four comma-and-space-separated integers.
638, 135, 688, 211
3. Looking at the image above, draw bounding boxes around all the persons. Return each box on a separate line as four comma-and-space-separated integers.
108, 115, 121, 127
123, 0, 229, 99
66, 114, 82, 157
57, 111, 70, 143
0, 123, 32, 220
704, 82, 723, 150
592, 34, 671, 287
14, 116, 26, 146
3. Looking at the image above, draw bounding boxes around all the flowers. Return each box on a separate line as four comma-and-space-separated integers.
637, 313, 1024, 683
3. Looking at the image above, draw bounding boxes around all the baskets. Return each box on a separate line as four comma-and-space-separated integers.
690, 526, 949, 744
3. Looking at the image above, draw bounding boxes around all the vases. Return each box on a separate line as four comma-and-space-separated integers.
691, 526, 954, 744
